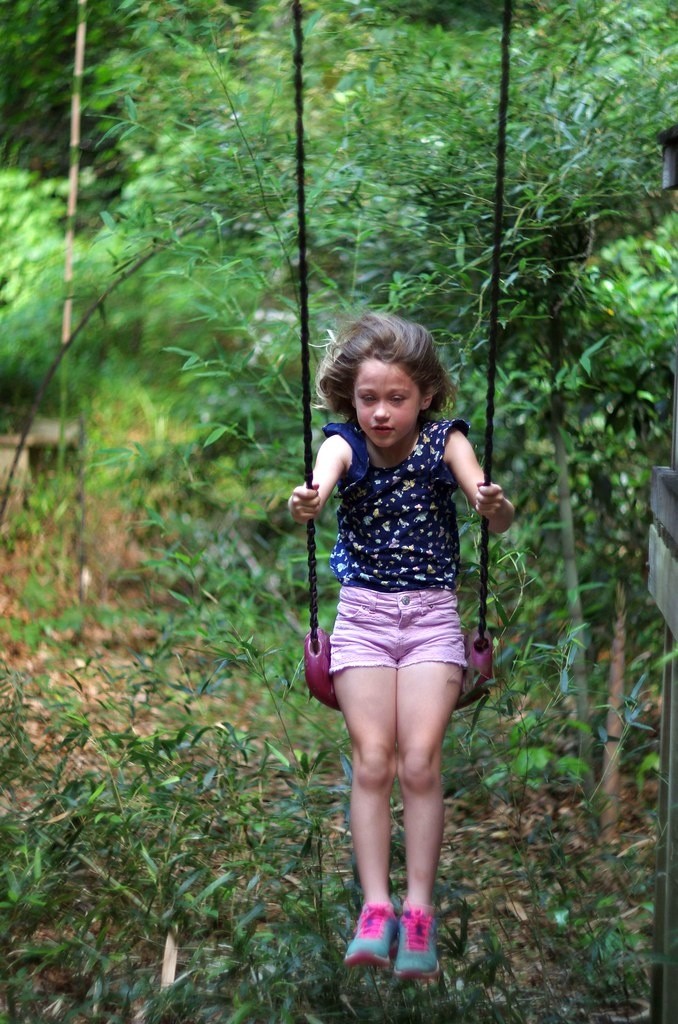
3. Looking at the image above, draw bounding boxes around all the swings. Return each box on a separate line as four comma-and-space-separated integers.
293, 0, 497, 714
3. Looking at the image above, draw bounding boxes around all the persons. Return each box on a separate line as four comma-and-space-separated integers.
289, 312, 515, 977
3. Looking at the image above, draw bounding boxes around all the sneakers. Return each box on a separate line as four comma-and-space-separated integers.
342, 900, 402, 969
394, 899, 441, 982
